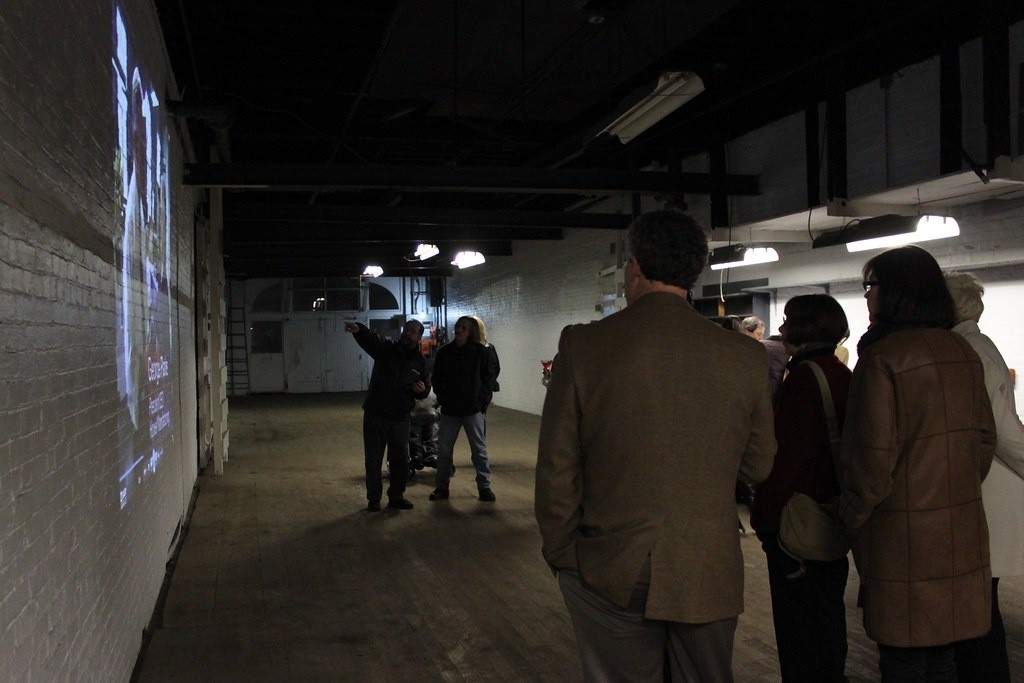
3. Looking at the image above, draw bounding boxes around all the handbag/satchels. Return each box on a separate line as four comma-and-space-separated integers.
774, 492, 852, 581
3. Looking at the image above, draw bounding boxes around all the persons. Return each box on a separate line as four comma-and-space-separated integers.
942, 270, 1024, 683
835, 330, 849, 365
429, 316, 500, 501
535, 211, 778, 683
750, 294, 853, 683
742, 316, 765, 340
836, 245, 997, 683
344, 319, 431, 512
723, 315, 745, 333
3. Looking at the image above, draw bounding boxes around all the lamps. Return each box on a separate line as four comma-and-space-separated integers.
708, 242, 779, 270
812, 212, 962, 254
595, 70, 706, 144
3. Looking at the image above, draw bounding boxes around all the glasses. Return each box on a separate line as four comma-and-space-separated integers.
863, 280, 880, 291
782, 316, 788, 327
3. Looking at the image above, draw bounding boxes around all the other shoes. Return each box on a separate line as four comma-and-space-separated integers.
388, 498, 414, 509
429, 485, 450, 500
478, 487, 496, 501
367, 500, 380, 512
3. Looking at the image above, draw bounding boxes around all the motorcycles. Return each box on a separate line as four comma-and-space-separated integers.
408, 386, 456, 483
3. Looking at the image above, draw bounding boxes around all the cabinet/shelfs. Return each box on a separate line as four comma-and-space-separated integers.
693, 291, 769, 338
